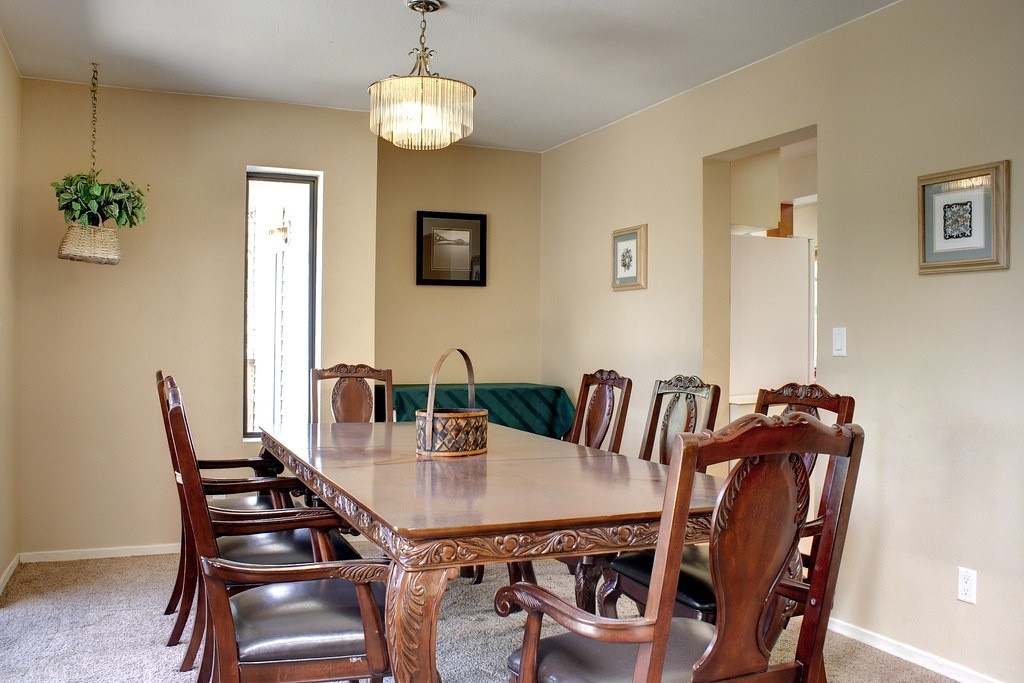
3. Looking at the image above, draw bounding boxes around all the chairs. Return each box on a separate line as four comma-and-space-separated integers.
311, 363, 393, 424
155, 370, 393, 683
472, 370, 864, 683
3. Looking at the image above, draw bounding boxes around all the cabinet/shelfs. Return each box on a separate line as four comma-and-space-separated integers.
731, 147, 780, 234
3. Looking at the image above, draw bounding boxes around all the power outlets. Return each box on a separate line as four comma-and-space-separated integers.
957, 567, 976, 604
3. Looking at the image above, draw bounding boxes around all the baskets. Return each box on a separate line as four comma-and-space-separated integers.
57, 210, 120, 264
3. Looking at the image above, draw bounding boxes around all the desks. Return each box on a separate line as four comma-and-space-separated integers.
375, 383, 576, 440
254, 420, 804, 683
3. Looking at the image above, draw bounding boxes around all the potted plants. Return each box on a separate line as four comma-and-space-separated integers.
49, 168, 150, 265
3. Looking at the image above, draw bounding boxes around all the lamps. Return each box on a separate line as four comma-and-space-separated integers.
368, 0, 476, 150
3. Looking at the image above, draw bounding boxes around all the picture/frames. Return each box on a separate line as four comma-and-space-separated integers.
611, 223, 647, 292
416, 210, 486, 286
918, 160, 1010, 275
